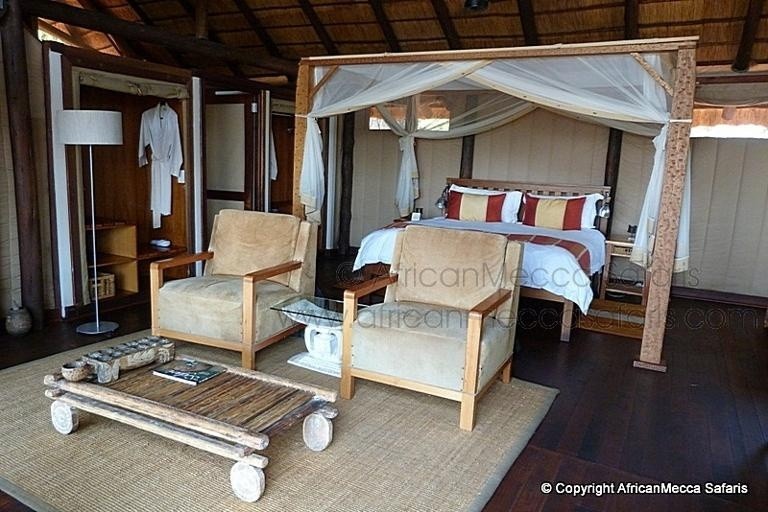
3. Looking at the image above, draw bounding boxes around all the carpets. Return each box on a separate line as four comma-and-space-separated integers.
575, 298, 647, 340
1, 328, 561, 512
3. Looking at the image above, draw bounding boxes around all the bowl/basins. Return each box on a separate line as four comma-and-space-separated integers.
61, 359, 90, 381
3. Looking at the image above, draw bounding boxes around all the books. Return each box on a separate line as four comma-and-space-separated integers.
151, 355, 226, 386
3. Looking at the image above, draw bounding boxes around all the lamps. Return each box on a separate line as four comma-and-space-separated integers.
56, 110, 124, 336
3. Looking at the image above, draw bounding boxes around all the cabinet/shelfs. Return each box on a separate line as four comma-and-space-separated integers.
42, 39, 205, 324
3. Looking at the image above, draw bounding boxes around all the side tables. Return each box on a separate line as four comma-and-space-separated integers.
269, 286, 384, 379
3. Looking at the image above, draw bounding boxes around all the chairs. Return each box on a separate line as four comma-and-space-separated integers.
339, 225, 525, 432
149, 209, 319, 370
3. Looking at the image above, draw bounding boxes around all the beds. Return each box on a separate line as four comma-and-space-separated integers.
42, 353, 339, 503
350, 177, 612, 342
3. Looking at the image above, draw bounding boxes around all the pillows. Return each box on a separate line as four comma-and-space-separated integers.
522, 192, 605, 229
522, 192, 586, 231
445, 190, 506, 223
448, 183, 522, 225
211, 209, 301, 288
395, 225, 509, 318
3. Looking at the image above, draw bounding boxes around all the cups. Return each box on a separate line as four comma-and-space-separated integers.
5, 308, 33, 338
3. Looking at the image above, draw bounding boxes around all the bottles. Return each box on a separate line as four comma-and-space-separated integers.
627, 225, 637, 241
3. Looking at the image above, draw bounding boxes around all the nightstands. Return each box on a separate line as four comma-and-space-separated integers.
599, 233, 651, 307
